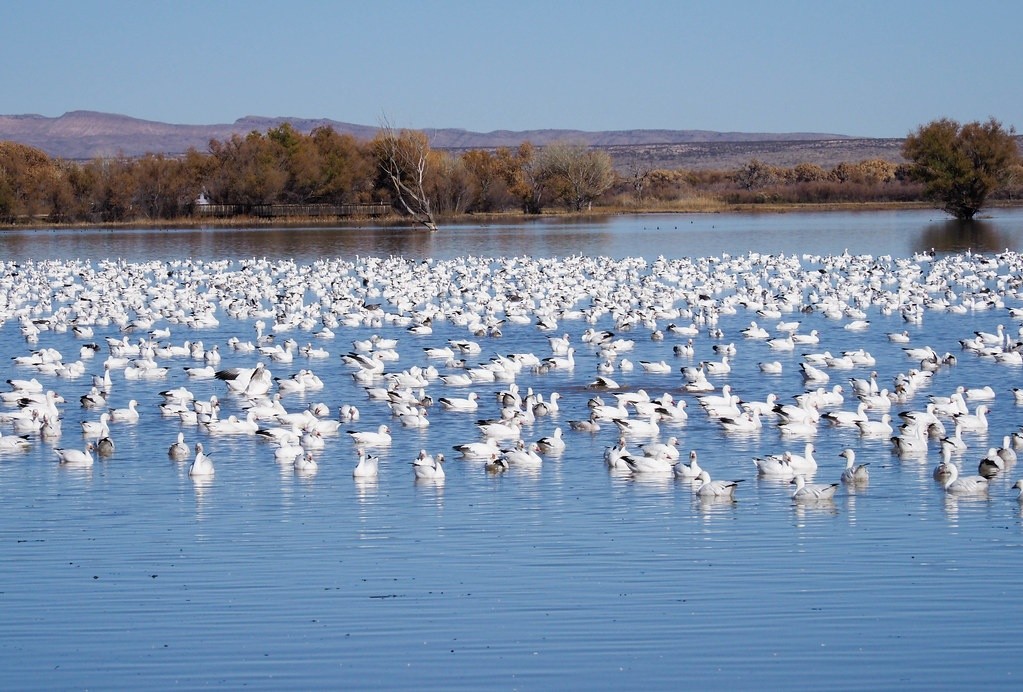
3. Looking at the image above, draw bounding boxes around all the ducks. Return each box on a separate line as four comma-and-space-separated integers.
0, 246, 1023, 503
